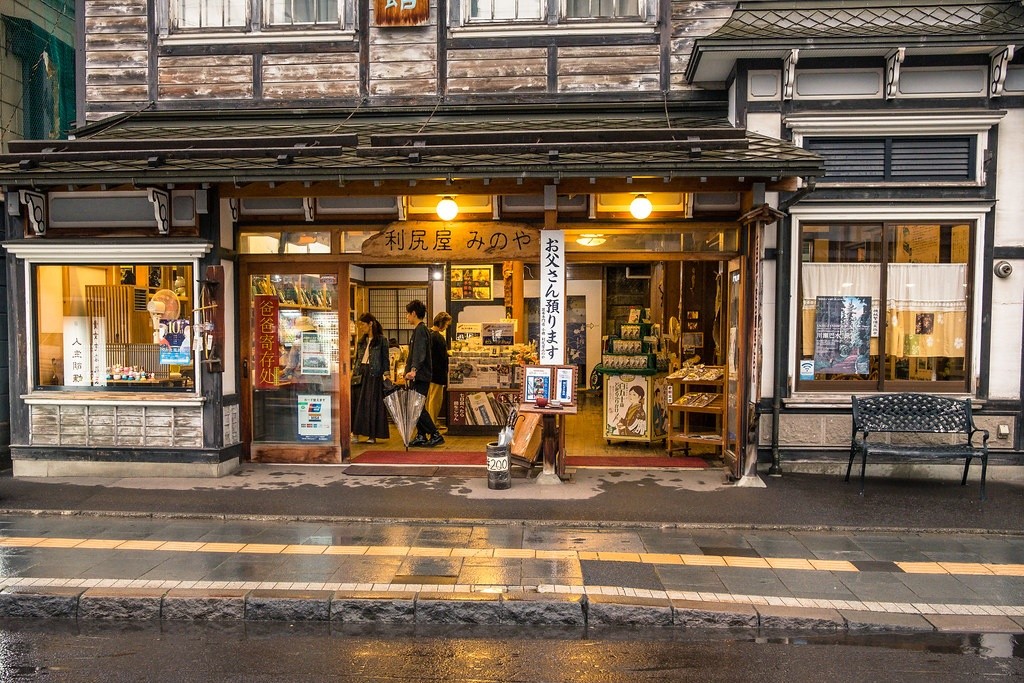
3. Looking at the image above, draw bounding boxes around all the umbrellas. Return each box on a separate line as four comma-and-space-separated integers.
383, 376, 426, 452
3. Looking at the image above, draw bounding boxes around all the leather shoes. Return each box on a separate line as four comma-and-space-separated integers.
422, 432, 444, 447
404, 432, 426, 447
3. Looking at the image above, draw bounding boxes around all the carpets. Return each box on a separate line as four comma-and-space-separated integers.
344, 465, 439, 477
434, 466, 543, 479
348, 450, 714, 468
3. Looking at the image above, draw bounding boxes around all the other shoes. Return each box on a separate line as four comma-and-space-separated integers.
366, 437, 376, 443
350, 437, 359, 443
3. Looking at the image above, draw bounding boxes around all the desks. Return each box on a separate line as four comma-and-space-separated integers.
108, 379, 167, 387
519, 404, 578, 485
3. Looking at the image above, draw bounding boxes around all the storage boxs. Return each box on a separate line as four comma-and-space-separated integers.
511, 412, 544, 460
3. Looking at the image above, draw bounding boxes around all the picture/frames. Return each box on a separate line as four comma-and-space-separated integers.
556, 365, 578, 406
683, 348, 695, 354
523, 365, 556, 405
681, 332, 705, 349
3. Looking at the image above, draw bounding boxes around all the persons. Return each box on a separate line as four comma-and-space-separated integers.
350, 312, 391, 444
403, 299, 446, 449
425, 312, 452, 439
279, 316, 323, 444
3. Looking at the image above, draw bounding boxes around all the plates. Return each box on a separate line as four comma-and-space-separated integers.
549, 405, 563, 407
534, 406, 550, 409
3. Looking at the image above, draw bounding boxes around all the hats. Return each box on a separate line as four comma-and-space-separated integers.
292, 316, 316, 331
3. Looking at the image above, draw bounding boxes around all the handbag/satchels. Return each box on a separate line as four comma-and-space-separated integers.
351, 375, 362, 385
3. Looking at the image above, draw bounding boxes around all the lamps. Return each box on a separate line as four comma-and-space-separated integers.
435, 194, 462, 221
628, 192, 653, 220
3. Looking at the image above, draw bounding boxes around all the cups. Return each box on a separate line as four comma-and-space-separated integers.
536, 398, 548, 407
551, 400, 561, 406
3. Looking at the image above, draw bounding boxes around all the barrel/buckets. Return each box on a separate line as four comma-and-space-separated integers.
486, 441, 512, 490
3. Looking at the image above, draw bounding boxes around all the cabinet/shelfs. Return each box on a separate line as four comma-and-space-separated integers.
252, 274, 358, 359
665, 366, 726, 458
595, 323, 672, 448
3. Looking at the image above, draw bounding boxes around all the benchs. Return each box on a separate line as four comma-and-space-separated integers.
843, 393, 989, 501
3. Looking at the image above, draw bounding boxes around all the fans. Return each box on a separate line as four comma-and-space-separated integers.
147, 289, 181, 344
